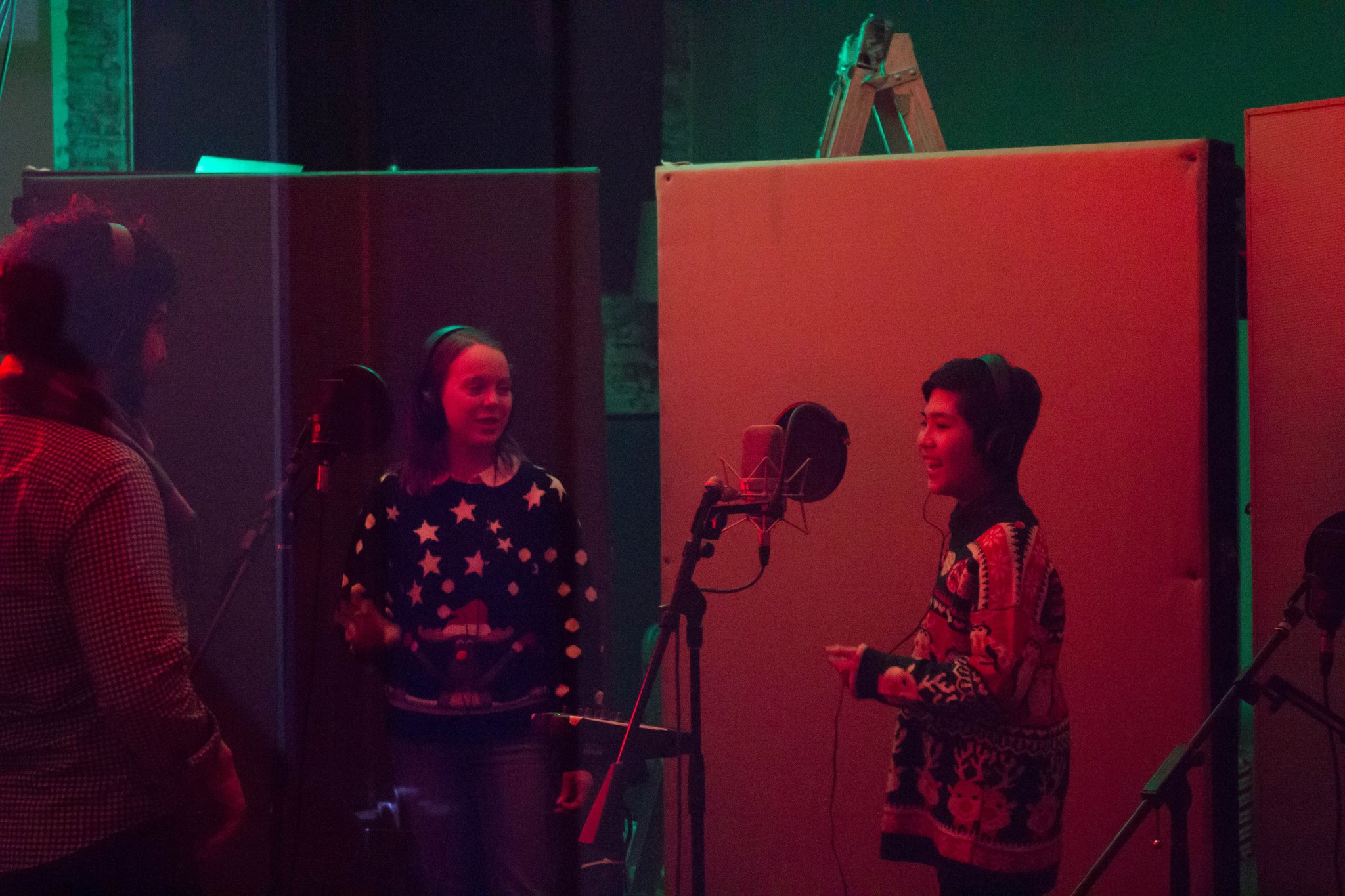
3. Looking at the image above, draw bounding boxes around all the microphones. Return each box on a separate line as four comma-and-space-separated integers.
311, 378, 344, 494
1310, 575, 1341, 672
741, 422, 789, 568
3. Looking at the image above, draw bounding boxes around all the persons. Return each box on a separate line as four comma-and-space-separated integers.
333, 326, 605, 896
826, 352, 1073, 896
0, 194, 250, 896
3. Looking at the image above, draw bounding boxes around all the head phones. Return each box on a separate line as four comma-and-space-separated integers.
977, 353, 1022, 469
84, 223, 137, 369
413, 324, 473, 428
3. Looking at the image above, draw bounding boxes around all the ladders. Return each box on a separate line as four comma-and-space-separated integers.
809, 15, 952, 160
